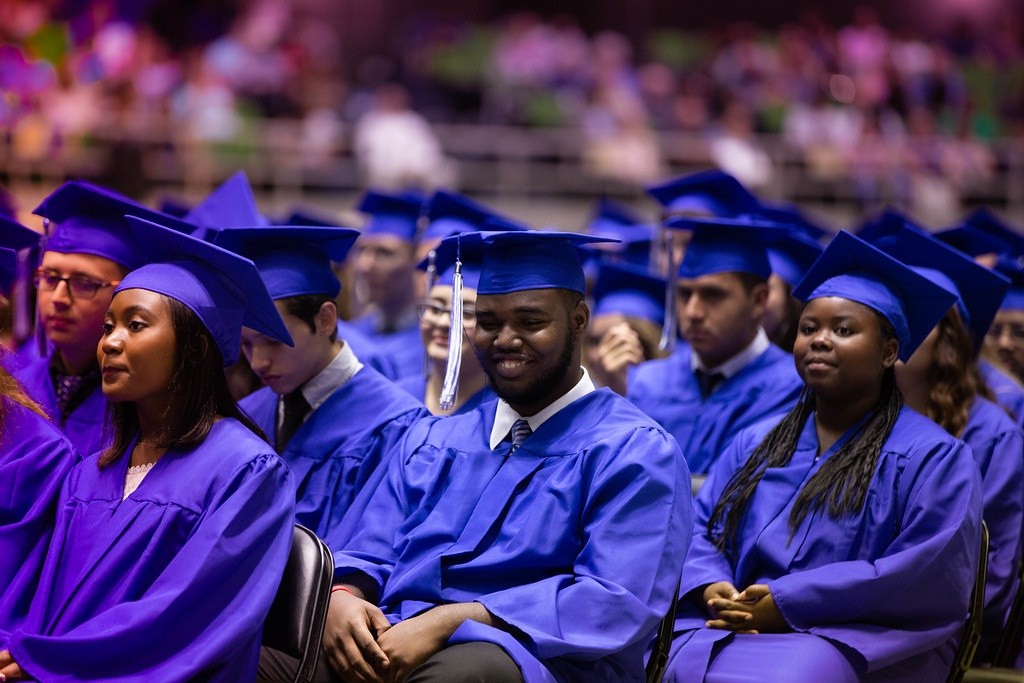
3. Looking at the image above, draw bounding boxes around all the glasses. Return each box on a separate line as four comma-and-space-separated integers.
31, 268, 122, 299
416, 304, 478, 330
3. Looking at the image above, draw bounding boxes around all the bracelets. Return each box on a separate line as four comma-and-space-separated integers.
332, 587, 351, 594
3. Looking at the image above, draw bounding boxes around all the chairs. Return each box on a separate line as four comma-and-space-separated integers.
251, 523, 342, 682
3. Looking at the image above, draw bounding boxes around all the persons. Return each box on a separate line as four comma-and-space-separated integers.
0, 167, 1024, 683
0, 0, 1006, 221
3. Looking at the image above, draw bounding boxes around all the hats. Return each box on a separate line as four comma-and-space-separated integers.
0, 213, 43, 290
32, 182, 200, 270
181, 170, 265, 240
888, 224, 1012, 357
791, 230, 960, 366
438, 231, 623, 411
658, 219, 787, 354
111, 215, 295, 368
356, 167, 1024, 324
212, 225, 361, 300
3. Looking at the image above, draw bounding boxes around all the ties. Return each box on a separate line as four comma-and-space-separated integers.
506, 420, 531, 458
696, 371, 726, 402
54, 370, 99, 415
276, 389, 312, 458
